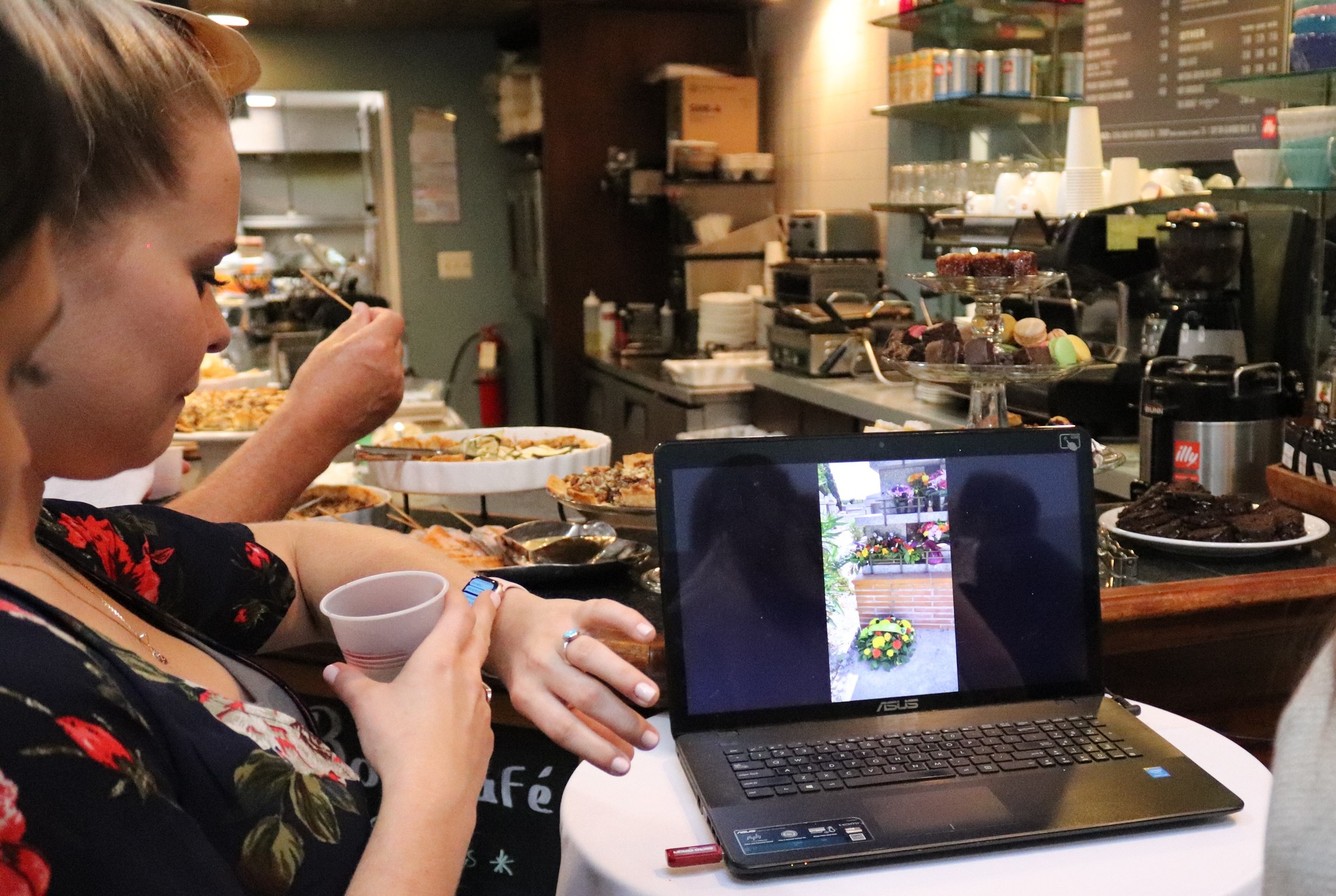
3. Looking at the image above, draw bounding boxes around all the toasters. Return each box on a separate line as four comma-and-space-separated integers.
786, 210, 881, 260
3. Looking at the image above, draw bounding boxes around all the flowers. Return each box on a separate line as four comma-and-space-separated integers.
849, 518, 950, 575
849, 615, 917, 672
882, 469, 948, 503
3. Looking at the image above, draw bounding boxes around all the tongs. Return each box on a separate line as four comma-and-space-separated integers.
1096, 525, 1138, 579
355, 435, 505, 457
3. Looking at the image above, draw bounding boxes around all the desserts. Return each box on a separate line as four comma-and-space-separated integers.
1116, 473, 1306, 542
937, 251, 1038, 278
881, 313, 1094, 364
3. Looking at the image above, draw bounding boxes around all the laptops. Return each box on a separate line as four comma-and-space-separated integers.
652, 428, 1244, 881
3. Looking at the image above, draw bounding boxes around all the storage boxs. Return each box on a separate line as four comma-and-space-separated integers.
665, 75, 760, 165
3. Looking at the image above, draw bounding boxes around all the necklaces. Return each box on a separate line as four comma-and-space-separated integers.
0, 548, 168, 665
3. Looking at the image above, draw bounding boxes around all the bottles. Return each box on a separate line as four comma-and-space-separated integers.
602, 303, 619, 354
1281, 346, 1336, 488
582, 289, 601, 356
660, 300, 676, 342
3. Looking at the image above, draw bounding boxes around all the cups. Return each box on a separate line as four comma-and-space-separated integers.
890, 159, 1066, 206
965, 107, 1233, 219
747, 240, 786, 347
143, 446, 183, 504
318, 571, 449, 683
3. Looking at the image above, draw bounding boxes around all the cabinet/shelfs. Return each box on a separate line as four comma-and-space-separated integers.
868, 0, 1086, 214
233, 93, 375, 269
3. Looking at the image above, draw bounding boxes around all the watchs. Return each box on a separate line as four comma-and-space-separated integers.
457, 574, 528, 679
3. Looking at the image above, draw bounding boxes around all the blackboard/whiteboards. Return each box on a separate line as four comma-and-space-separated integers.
1082, 0, 1291, 163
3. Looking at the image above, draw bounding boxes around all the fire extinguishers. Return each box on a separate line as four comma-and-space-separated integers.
444, 322, 510, 428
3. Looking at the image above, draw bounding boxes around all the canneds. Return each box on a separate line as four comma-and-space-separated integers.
934, 47, 1035, 102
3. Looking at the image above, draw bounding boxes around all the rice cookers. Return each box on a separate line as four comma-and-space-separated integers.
1128, 356, 1284, 499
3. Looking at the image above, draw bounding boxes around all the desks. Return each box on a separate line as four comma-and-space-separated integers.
556, 693, 1272, 896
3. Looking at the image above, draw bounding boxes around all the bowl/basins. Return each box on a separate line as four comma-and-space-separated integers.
672, 139, 775, 183
1234, 104, 1336, 186
311, 483, 392, 530
1287, 0, 1336, 68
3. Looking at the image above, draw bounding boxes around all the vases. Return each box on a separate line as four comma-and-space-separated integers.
928, 549, 943, 565
894, 502, 908, 514
930, 496, 948, 511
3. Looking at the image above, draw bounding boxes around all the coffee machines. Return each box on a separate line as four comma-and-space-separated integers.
1140, 215, 1249, 365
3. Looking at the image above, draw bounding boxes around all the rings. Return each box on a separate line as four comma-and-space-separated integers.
562, 630, 588, 665
483, 680, 492, 703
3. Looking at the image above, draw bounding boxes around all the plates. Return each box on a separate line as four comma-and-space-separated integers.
1097, 506, 1330, 555
696, 292, 767, 359
545, 485, 657, 513
172, 372, 613, 494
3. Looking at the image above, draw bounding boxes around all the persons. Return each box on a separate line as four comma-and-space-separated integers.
1264, 628, 1336, 896
118, 297, 407, 524
0, 1, 675, 896
